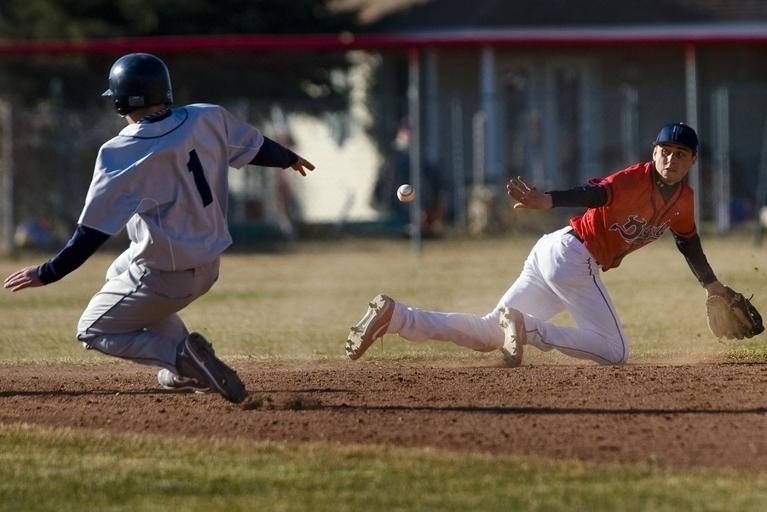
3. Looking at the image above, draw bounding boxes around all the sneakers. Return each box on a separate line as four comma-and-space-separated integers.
344, 292, 395, 361
497, 306, 525, 367
157, 368, 214, 393
177, 331, 246, 403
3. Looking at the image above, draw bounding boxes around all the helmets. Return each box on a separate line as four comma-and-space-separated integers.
101, 52, 174, 115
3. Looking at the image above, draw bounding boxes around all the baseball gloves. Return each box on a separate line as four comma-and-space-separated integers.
706, 286, 765, 340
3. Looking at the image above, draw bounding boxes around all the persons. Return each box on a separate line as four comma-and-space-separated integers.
345, 120, 740, 370
4, 51, 317, 403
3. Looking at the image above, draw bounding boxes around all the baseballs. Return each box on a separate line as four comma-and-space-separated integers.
397, 184, 416, 203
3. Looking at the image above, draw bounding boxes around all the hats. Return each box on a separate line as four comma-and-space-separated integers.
652, 121, 698, 152
